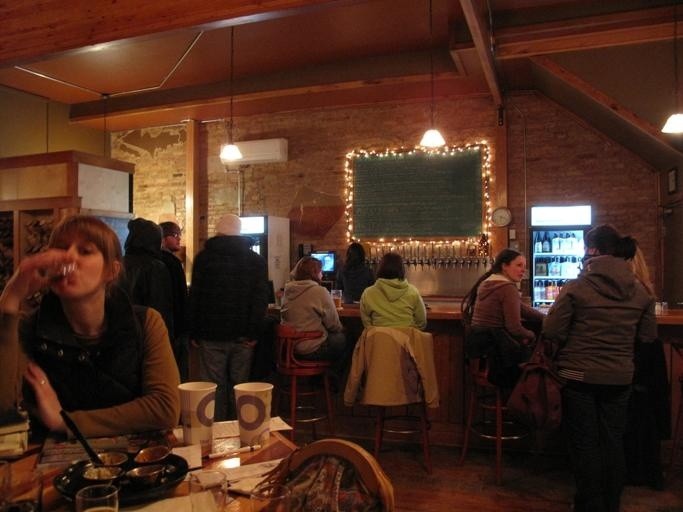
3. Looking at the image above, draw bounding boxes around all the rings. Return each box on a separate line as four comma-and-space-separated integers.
39, 380, 45, 386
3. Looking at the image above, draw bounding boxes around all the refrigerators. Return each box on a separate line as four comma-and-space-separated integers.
528, 203, 594, 313
238, 215, 290, 311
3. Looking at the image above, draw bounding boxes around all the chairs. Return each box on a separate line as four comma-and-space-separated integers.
267, 436, 396, 512
374, 402, 433, 476
270, 324, 338, 444
458, 355, 533, 486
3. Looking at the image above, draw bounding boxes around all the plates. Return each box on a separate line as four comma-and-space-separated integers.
53, 453, 189, 505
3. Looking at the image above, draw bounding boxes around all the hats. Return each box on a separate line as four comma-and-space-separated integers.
127, 218, 161, 247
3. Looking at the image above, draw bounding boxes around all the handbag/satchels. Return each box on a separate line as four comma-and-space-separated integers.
506, 342, 562, 431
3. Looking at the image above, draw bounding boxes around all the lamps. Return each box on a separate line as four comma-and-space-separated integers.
419, 0, 445, 148
220, 26, 243, 160
660, 0, 683, 134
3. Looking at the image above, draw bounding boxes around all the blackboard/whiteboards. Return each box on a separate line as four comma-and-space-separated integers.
352, 152, 483, 237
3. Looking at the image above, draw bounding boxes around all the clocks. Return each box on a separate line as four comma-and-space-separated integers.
666, 165, 678, 195
492, 207, 512, 228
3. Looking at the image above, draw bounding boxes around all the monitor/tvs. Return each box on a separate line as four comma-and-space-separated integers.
310, 251, 337, 280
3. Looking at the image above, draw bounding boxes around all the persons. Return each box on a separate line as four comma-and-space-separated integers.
335, 243, 373, 304
0, 216, 181, 442
541, 223, 671, 512
360, 253, 428, 330
119, 218, 267, 421
279, 255, 347, 394
461, 248, 546, 388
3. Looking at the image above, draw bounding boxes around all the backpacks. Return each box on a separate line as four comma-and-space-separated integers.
466, 331, 519, 388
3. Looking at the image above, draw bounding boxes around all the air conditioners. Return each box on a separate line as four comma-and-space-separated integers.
219, 139, 289, 166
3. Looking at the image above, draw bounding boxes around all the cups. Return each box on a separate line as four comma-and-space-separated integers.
233, 382, 274, 448
250, 484, 291, 512
331, 288, 342, 309
178, 382, 217, 458
74, 484, 119, 512
189, 471, 228, 512
53, 258, 78, 281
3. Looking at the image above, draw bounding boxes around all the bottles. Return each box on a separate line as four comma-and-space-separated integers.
534, 231, 583, 254
535, 256, 582, 276
535, 279, 566, 302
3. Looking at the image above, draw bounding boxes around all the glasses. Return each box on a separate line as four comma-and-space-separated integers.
167, 233, 179, 240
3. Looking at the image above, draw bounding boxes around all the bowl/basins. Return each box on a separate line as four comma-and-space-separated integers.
77, 445, 173, 503
